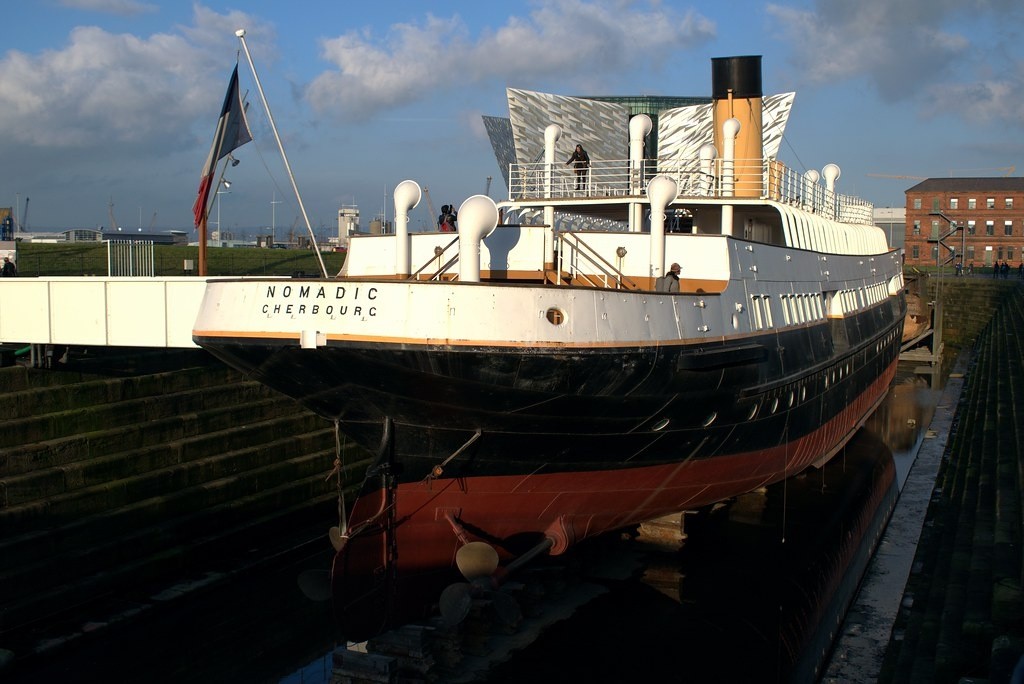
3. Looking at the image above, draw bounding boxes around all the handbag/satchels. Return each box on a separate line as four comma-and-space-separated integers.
439, 213, 455, 232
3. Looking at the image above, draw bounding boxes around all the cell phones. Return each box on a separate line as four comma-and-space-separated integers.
450, 204, 452, 213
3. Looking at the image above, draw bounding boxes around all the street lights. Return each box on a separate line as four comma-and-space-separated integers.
269, 192, 283, 244
217, 192, 230, 247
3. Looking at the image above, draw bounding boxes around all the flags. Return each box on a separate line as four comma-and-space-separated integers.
190, 49, 254, 229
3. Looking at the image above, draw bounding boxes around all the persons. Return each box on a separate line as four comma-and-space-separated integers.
993, 261, 1024, 279
438, 204, 458, 233
564, 144, 591, 192
662, 262, 683, 293
0, 258, 16, 277
954, 261, 974, 277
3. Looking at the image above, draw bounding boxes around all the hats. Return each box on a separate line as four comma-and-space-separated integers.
671, 263, 681, 272
441, 204, 449, 213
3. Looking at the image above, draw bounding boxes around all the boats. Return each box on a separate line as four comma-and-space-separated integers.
193, 55, 910, 597
